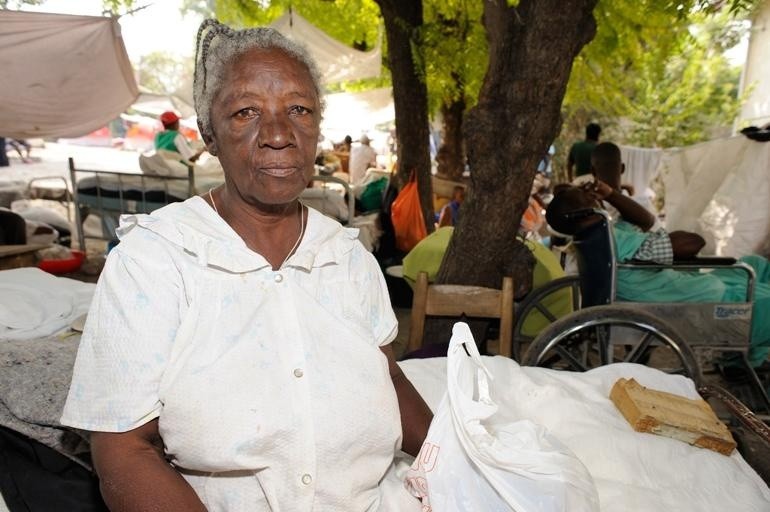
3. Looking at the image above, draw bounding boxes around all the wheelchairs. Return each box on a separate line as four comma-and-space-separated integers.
508, 203, 770, 424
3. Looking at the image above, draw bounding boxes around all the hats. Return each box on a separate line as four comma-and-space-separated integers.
160, 112, 183, 126
361, 136, 373, 143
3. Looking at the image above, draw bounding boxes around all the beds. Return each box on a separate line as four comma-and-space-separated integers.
68, 157, 355, 262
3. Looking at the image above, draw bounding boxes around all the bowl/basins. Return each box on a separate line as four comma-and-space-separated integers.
39, 249, 84, 274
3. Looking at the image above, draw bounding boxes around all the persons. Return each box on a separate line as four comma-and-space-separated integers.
543, 176, 770, 383
438, 185, 463, 227
155, 110, 200, 169
403, 221, 583, 371
566, 122, 603, 181
552, 139, 662, 232
59, 16, 433, 510
348, 134, 377, 185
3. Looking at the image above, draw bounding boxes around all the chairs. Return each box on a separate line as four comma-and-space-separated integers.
404, 271, 514, 366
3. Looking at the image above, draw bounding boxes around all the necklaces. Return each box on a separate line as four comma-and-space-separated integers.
208, 188, 307, 274
